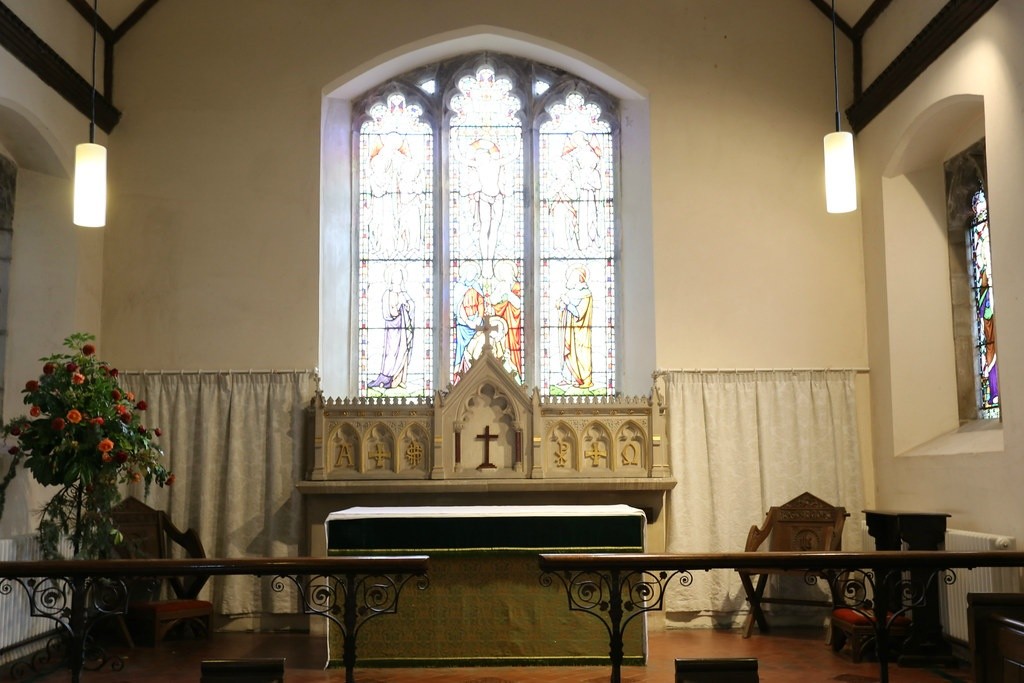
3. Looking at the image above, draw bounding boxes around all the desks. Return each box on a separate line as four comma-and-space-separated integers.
324, 504, 646, 668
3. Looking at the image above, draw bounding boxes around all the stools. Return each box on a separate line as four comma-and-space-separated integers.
830, 609, 910, 663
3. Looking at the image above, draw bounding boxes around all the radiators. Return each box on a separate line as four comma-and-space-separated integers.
941, 529, 1019, 642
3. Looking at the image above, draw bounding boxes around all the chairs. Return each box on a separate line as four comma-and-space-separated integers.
735, 492, 851, 645
98, 496, 213, 647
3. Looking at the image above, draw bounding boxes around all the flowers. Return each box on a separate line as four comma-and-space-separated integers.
0, 333, 175, 559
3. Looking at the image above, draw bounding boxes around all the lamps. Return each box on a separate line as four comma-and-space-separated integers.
72, 0, 107, 227
823, 0, 857, 213
